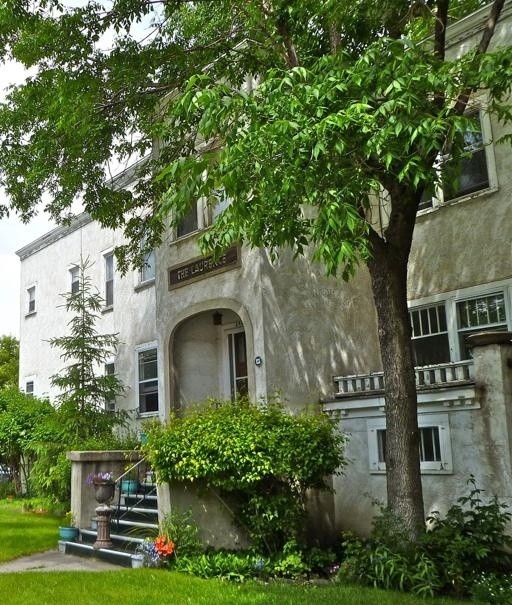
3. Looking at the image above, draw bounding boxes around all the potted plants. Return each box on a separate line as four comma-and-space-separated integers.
145, 458, 155, 483
58, 512, 79, 541
6, 484, 15, 503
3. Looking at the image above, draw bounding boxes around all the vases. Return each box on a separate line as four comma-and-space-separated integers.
131, 555, 144, 569
122, 481, 138, 493
94, 486, 113, 503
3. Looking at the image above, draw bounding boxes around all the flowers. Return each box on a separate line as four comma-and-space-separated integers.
87, 472, 112, 485
135, 538, 160, 564
124, 463, 136, 479
